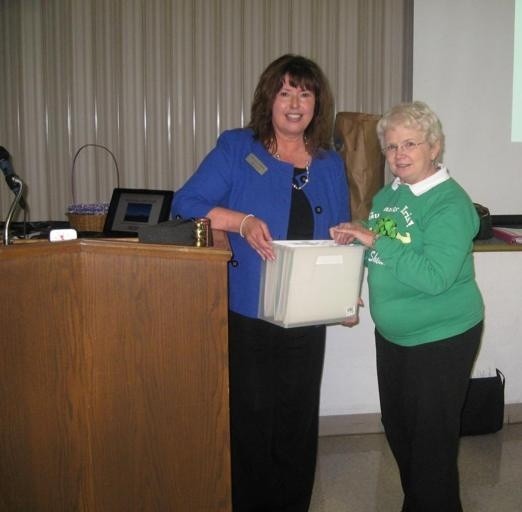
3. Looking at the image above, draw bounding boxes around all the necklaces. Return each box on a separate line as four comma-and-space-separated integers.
270, 135, 309, 191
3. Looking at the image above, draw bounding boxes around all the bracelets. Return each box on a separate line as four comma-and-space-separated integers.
239, 214, 255, 239
371, 233, 377, 248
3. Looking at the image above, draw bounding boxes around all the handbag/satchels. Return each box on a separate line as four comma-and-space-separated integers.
458, 369, 505, 437
335, 112, 385, 222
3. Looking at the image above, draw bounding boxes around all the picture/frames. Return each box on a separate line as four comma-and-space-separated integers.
103, 188, 173, 237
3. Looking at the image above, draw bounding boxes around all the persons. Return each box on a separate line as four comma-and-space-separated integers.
328, 99, 485, 511
171, 53, 363, 511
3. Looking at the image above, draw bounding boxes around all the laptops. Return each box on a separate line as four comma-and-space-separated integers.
76, 188, 174, 239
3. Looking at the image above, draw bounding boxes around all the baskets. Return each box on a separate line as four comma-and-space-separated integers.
65, 145, 120, 232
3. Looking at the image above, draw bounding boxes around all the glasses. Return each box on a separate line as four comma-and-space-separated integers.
383, 139, 424, 153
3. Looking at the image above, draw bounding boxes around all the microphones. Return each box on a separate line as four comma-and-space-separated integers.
0, 146, 25, 209
